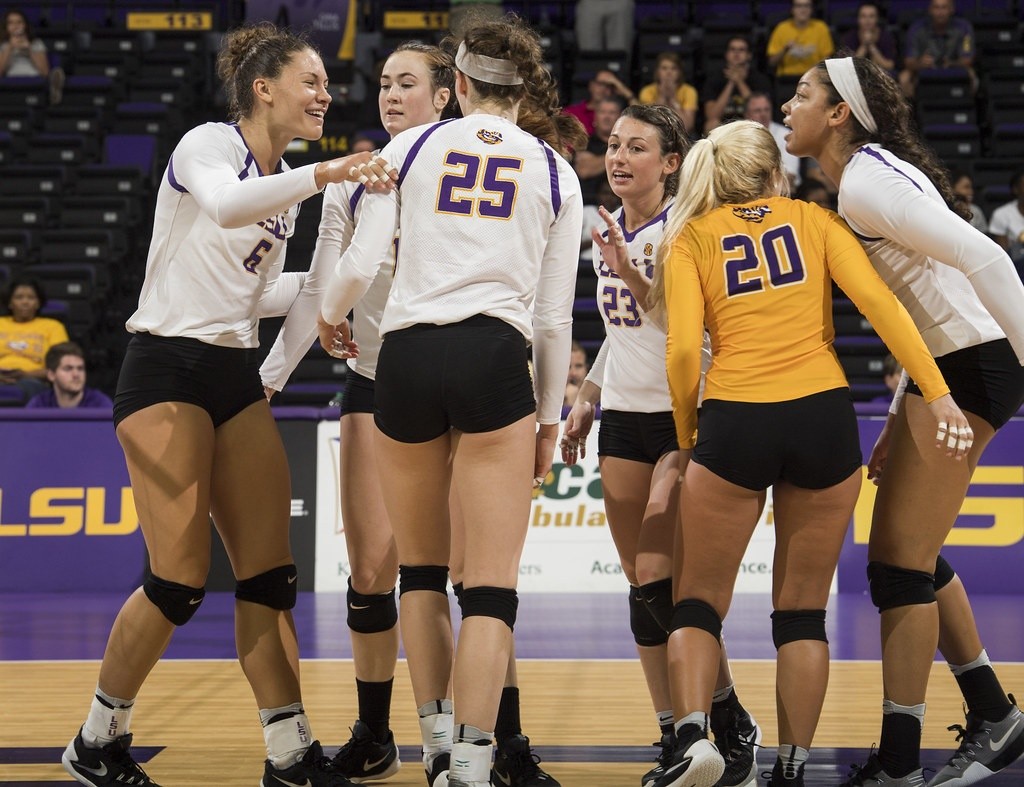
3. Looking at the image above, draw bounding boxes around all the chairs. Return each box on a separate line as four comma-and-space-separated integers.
0, 16, 1024, 406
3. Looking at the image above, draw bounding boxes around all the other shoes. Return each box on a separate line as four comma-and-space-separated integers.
421, 746, 450, 787
445, 742, 496, 787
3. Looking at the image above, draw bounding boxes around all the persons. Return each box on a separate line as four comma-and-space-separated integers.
780, 55, 1024, 787
0, 9, 65, 106
526, 0, 1024, 406
62, 21, 399, 787
643, 120, 974, 787
315, 19, 584, 787
260, 42, 560, 787
24, 341, 113, 408
0, 277, 70, 386
560, 104, 763, 787
347, 135, 376, 154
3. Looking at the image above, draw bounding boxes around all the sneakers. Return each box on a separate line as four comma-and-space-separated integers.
837, 742, 926, 787
326, 718, 401, 781
642, 724, 677, 785
642, 732, 725, 787
260, 738, 365, 787
60, 722, 161, 787
710, 688, 766, 787
491, 734, 561, 787
761, 755, 806, 787
922, 693, 1024, 787
